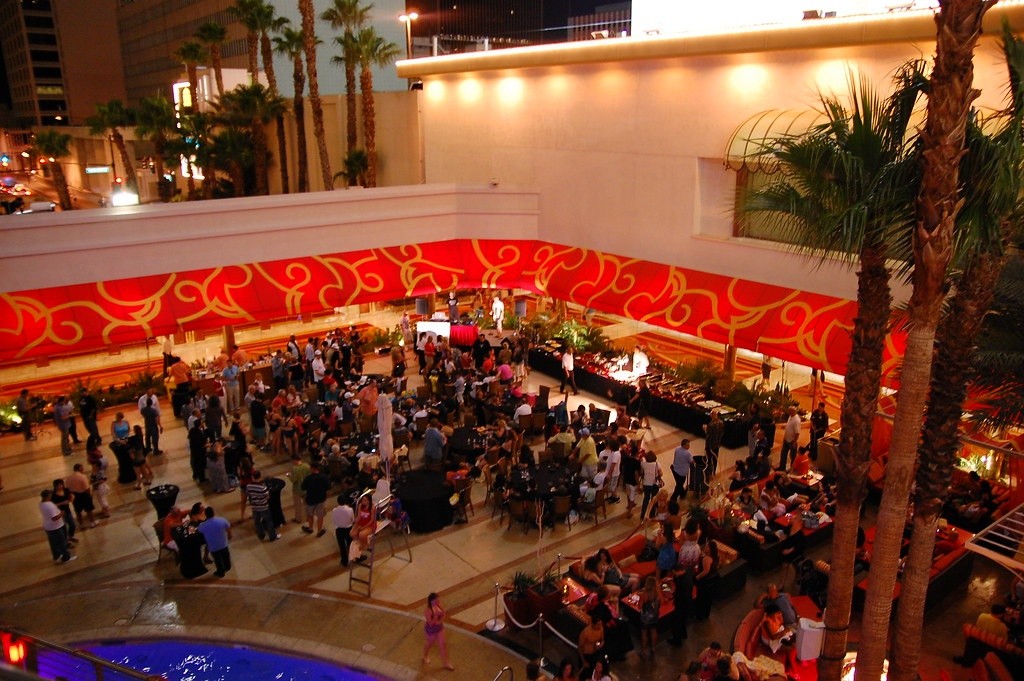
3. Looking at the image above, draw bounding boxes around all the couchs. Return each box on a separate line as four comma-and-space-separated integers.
556, 464, 1023, 681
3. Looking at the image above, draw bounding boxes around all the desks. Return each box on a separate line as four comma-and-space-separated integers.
530, 335, 754, 449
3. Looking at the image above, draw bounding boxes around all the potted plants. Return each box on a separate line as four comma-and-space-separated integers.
529, 566, 560, 625
501, 571, 537, 635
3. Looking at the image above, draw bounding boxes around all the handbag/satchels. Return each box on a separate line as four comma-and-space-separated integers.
617, 555, 636, 569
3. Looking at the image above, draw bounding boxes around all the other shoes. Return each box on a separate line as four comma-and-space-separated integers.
260, 535, 270, 542
666, 634, 687, 648
203, 557, 212, 564
62, 450, 72, 456
290, 518, 300, 524
55, 556, 76, 564
96, 511, 110, 520
351, 555, 368, 564
340, 560, 347, 567
443, 664, 455, 670
271, 534, 282, 540
78, 524, 94, 531
65, 536, 78, 549
26, 435, 36, 442
182, 332, 839, 516
213, 572, 224, 579
640, 648, 657, 660
421, 654, 430, 666
144, 449, 162, 456
235, 518, 244, 524
315, 531, 324, 537
301, 526, 312, 534
89, 449, 102, 459
72, 440, 81, 444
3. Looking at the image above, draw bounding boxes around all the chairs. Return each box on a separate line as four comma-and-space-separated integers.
150, 316, 647, 562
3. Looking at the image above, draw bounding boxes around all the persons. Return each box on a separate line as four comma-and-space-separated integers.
12, 288, 1006, 681
